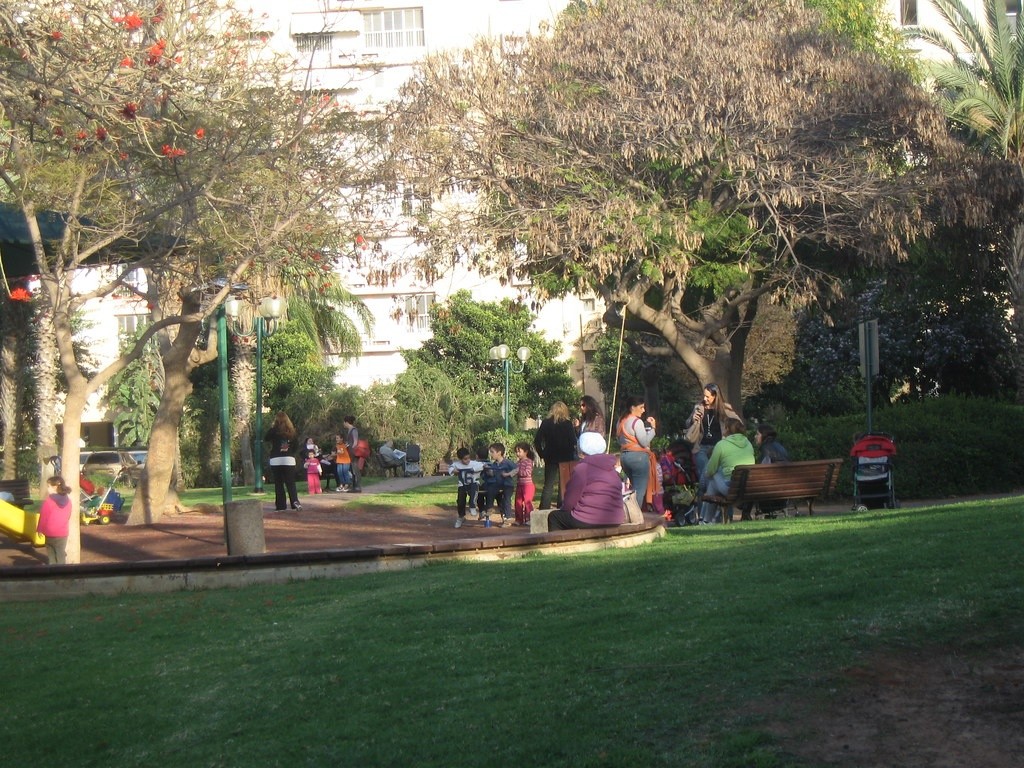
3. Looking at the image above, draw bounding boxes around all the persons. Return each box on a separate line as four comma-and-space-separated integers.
547, 432, 626, 533
380, 440, 408, 477
299, 437, 339, 489
616, 398, 656, 510
344, 416, 362, 493
36, 476, 72, 564
752, 425, 788, 520
534, 401, 577, 511
685, 383, 755, 526
613, 448, 676, 522
574, 395, 606, 462
303, 449, 323, 494
264, 412, 303, 512
327, 433, 353, 493
448, 443, 535, 529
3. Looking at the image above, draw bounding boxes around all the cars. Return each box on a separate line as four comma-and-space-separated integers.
55, 450, 148, 481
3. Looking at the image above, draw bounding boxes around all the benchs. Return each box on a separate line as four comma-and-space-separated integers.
438, 463, 459, 477
376, 451, 405, 478
0, 478, 33, 510
702, 457, 844, 522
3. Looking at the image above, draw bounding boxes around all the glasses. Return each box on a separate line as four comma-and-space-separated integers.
580, 404, 585, 408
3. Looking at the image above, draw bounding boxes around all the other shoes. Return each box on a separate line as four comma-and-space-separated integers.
699, 521, 712, 526
350, 487, 361, 492
294, 501, 302, 510
336, 483, 349, 492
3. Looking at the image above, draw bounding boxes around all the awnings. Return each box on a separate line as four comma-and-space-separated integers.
0, 200, 205, 279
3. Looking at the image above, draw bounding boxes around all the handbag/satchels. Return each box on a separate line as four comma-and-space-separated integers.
685, 417, 700, 443
547, 438, 574, 460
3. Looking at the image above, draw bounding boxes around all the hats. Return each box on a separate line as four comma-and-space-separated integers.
579, 432, 607, 455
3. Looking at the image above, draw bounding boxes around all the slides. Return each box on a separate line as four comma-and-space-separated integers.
0, 498, 46, 548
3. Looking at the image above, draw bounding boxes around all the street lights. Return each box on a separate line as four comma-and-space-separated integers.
206, 291, 286, 545
488, 344, 531, 434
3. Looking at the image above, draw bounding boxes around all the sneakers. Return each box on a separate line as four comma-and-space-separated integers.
478, 510, 490, 521
455, 517, 466, 528
525, 521, 530, 525
469, 507, 477, 516
514, 521, 524, 525
502, 518, 513, 528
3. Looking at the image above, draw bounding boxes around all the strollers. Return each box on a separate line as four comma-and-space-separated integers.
851, 430, 900, 512
405, 442, 424, 478
78, 468, 127, 525
660, 437, 699, 527
